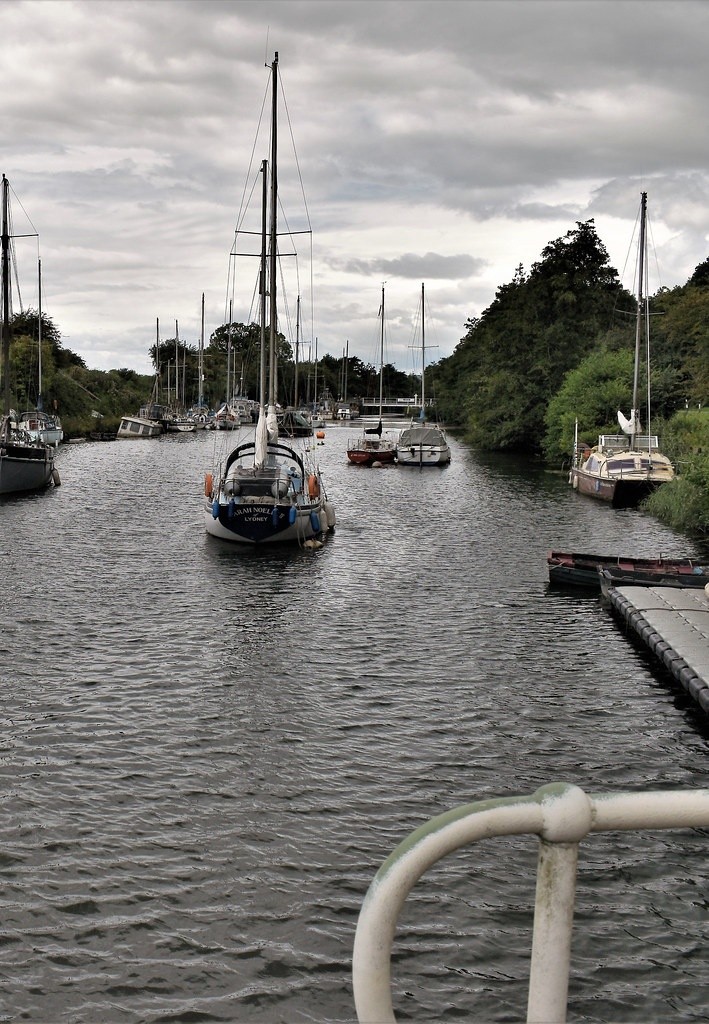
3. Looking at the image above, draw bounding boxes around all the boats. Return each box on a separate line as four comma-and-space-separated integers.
547, 552, 709, 589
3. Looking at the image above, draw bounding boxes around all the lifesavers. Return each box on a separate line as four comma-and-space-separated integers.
309, 476, 318, 499
577, 443, 590, 462
204, 473, 213, 497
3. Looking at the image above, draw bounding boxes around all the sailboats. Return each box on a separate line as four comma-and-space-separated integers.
0, 173, 63, 494
397, 283, 452, 463
346, 282, 397, 463
202, 24, 336, 544
566, 190, 675, 502
116, 292, 360, 437
